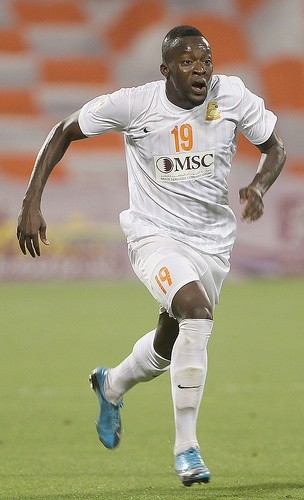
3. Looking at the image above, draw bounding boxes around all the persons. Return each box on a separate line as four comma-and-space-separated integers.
16, 25, 286, 487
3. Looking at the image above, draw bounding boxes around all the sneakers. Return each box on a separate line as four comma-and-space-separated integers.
175, 447, 211, 487
88, 367, 124, 449
